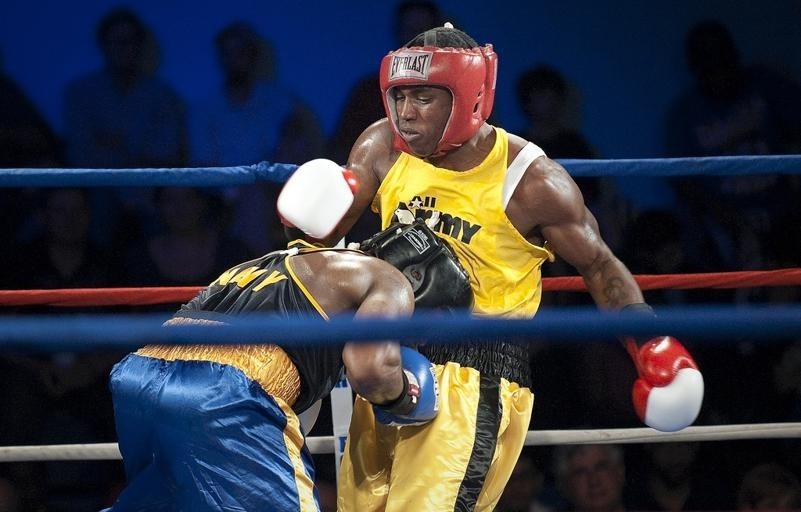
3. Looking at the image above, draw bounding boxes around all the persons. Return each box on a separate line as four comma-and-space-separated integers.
0, 0, 801, 512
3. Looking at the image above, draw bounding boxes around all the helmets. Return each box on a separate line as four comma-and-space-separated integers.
380, 43, 499, 161
358, 218, 475, 318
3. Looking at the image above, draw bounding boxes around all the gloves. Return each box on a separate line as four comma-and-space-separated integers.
624, 335, 707, 431
275, 158, 361, 241
372, 346, 439, 426
